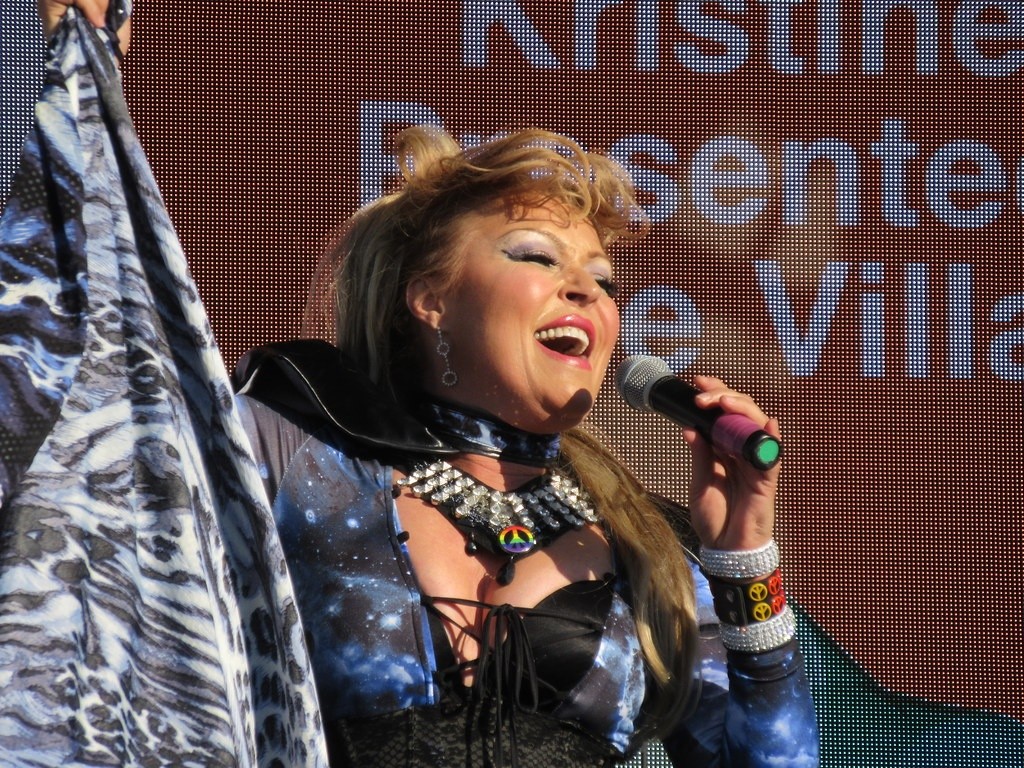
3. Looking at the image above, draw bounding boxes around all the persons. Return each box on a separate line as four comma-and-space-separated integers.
36, 1, 820, 768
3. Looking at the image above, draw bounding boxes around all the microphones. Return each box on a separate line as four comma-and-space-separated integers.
615, 355, 781, 470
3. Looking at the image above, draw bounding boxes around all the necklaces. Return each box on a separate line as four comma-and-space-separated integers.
393, 441, 602, 587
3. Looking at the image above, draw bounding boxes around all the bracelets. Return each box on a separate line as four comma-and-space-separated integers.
699, 541, 797, 652
